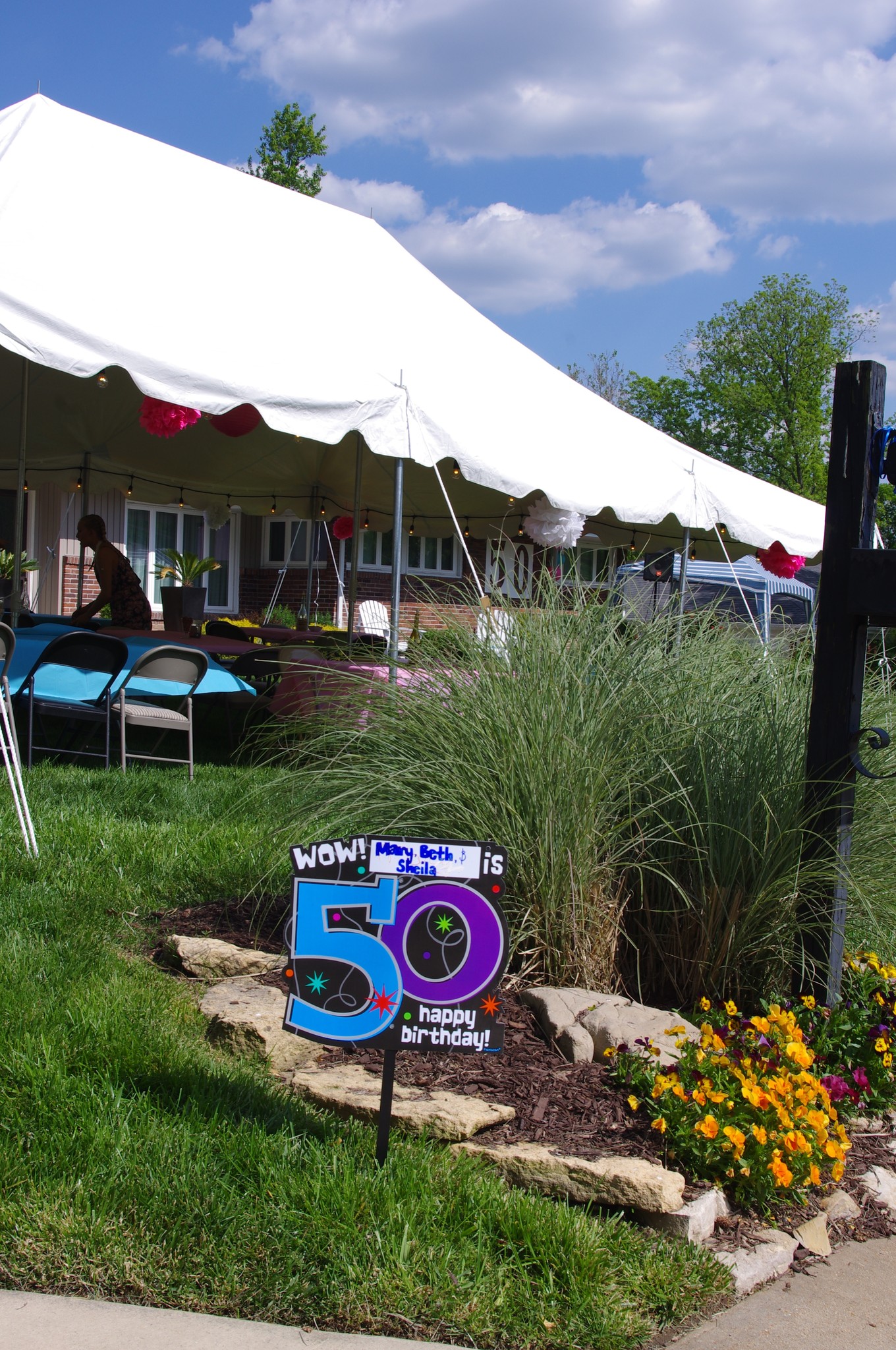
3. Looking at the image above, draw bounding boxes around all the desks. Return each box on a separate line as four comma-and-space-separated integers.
6, 610, 111, 631
242, 623, 378, 648
101, 626, 273, 672
264, 660, 521, 739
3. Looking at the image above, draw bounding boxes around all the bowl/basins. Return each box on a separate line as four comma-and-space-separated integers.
308, 625, 323, 632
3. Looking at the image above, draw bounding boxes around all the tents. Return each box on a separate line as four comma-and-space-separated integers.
0, 86, 887, 864
616, 550, 816, 670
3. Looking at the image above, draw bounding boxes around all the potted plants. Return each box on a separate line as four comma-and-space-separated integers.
151, 545, 222, 634
0, 549, 41, 611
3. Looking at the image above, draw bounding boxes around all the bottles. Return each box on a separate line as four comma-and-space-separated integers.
405, 613, 421, 667
295, 594, 308, 632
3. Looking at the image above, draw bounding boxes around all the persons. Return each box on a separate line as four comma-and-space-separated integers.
70, 514, 153, 631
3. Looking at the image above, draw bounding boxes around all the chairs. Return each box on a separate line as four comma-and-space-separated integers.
278, 646, 328, 679
192, 651, 286, 754
261, 624, 290, 647
0, 620, 25, 775
2, 612, 35, 630
205, 619, 268, 682
353, 633, 388, 656
8, 629, 129, 773
315, 631, 351, 658
107, 644, 209, 781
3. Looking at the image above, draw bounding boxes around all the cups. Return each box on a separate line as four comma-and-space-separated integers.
181, 617, 205, 639
